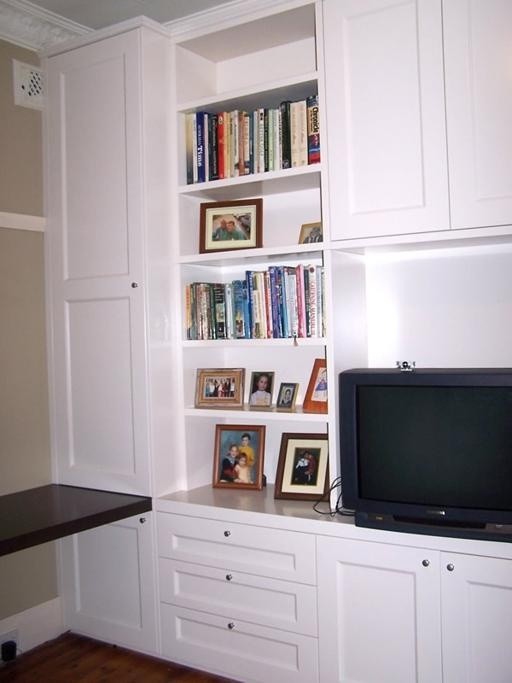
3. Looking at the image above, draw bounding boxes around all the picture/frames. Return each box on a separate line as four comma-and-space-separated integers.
302, 357, 329, 414
247, 370, 275, 408
195, 367, 246, 409
275, 381, 300, 411
273, 432, 330, 502
211, 423, 266, 491
298, 221, 323, 244
198, 197, 264, 254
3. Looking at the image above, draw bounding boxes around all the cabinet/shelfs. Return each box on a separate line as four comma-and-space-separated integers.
38, 17, 177, 659
318, 523, 512, 683
156, 510, 320, 683
313, 0, 512, 251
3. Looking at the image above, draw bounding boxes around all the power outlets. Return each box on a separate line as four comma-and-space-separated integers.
0, 627, 20, 663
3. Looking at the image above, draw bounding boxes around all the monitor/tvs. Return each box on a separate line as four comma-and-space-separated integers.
337, 367, 512, 543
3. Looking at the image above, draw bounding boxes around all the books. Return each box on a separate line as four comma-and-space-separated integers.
183, 90, 319, 183
185, 263, 323, 339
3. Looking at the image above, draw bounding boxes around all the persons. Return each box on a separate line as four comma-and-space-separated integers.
293, 454, 311, 482
220, 443, 239, 482
304, 451, 316, 476
236, 433, 255, 465
301, 225, 323, 243
205, 376, 235, 396
280, 387, 292, 406
249, 374, 270, 405
231, 451, 254, 484
225, 220, 246, 240
210, 219, 233, 240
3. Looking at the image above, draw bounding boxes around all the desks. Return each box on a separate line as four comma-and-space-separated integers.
0, 483, 153, 558
